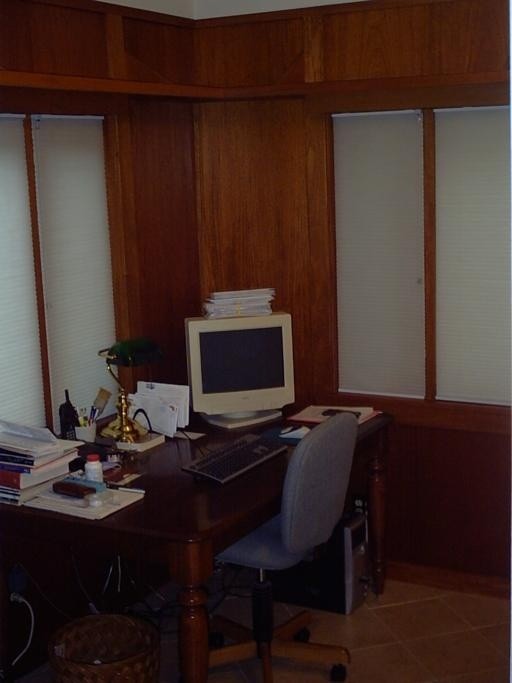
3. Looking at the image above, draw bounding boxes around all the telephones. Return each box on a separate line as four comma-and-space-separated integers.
59, 389, 80, 441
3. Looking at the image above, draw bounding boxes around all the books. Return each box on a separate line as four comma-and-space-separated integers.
287, 406, 378, 426
202, 288, 276, 320
0, 419, 145, 520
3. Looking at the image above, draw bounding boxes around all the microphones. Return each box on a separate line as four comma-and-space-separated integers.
107, 336, 154, 366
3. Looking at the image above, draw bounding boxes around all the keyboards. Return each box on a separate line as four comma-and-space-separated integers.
181, 433, 288, 484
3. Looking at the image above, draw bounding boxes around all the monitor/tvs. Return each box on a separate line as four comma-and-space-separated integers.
185, 311, 295, 431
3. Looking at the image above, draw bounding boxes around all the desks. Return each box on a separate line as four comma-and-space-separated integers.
0, 408, 395, 683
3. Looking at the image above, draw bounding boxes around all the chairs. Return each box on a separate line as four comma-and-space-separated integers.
208, 410, 361, 681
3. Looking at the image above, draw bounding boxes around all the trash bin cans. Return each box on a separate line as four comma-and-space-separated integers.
48, 613, 160, 683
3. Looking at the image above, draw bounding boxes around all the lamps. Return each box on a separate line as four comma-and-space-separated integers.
97, 336, 165, 445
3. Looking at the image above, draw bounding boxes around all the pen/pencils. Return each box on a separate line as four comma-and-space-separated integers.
73, 406, 99, 427
107, 485, 146, 494
